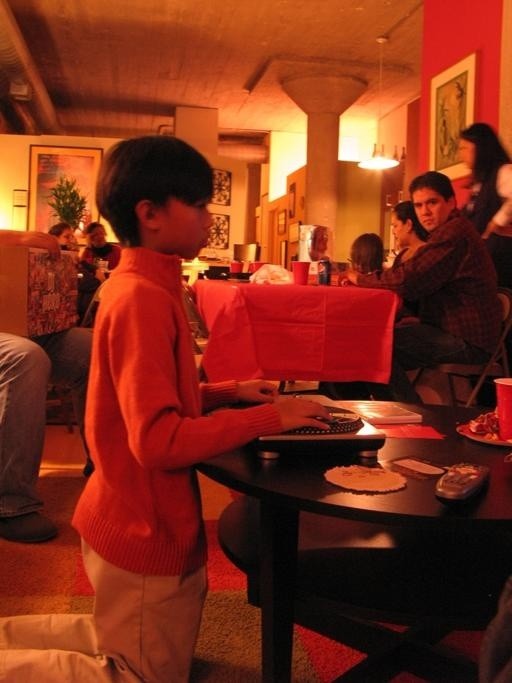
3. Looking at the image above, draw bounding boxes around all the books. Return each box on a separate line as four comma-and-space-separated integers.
339, 401, 423, 426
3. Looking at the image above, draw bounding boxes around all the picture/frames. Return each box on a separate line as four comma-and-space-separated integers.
288, 223, 299, 243
289, 182, 296, 219
428, 52, 478, 181
27, 144, 103, 247
276, 207, 286, 233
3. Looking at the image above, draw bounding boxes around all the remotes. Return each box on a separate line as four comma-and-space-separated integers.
434, 462, 490, 502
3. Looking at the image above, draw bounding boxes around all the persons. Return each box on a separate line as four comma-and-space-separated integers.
47, 222, 106, 314
391, 200, 427, 268
333, 171, 503, 408
351, 232, 383, 276
81, 222, 120, 276
457, 122, 512, 244
0, 325, 103, 544
0, 133, 332, 683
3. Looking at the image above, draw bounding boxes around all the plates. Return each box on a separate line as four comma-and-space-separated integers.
456, 421, 512, 445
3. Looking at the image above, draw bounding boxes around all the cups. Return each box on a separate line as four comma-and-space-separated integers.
229, 262, 243, 273
494, 378, 512, 441
99, 261, 108, 271
291, 261, 310, 285
249, 263, 268, 274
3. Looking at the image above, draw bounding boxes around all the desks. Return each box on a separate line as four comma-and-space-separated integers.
196, 279, 401, 399
191, 399, 512, 683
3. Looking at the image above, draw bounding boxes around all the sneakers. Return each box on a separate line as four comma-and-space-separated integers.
0, 512, 58, 542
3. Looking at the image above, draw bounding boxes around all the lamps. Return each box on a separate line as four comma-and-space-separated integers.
357, 38, 400, 170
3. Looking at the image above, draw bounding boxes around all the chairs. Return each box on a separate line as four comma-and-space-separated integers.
413, 288, 512, 406
